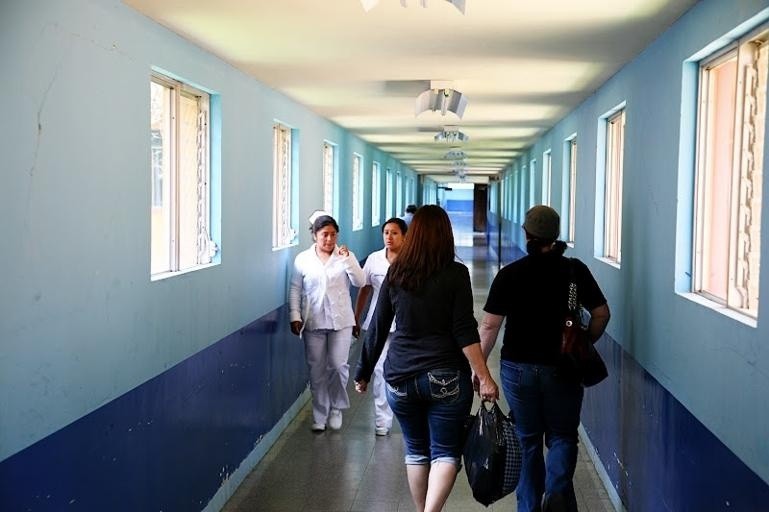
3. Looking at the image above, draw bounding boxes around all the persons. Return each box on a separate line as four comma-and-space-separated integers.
352, 217, 407, 436
401, 205, 417, 225
471, 205, 610, 511
353, 205, 500, 512
288, 210, 367, 432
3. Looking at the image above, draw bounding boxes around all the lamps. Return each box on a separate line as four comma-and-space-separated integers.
415, 89, 468, 179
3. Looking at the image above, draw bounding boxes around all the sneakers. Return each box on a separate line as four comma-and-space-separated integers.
375, 429, 389, 436
310, 422, 327, 432
328, 407, 343, 431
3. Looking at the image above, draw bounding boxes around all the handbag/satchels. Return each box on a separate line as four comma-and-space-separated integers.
552, 256, 609, 390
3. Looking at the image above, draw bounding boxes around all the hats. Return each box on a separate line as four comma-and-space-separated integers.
307, 209, 331, 225
521, 204, 561, 240
